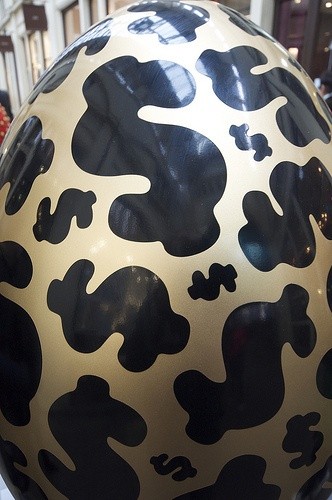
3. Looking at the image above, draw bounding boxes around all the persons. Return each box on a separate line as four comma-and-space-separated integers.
0, 90, 12, 146
318, 70, 332, 113
